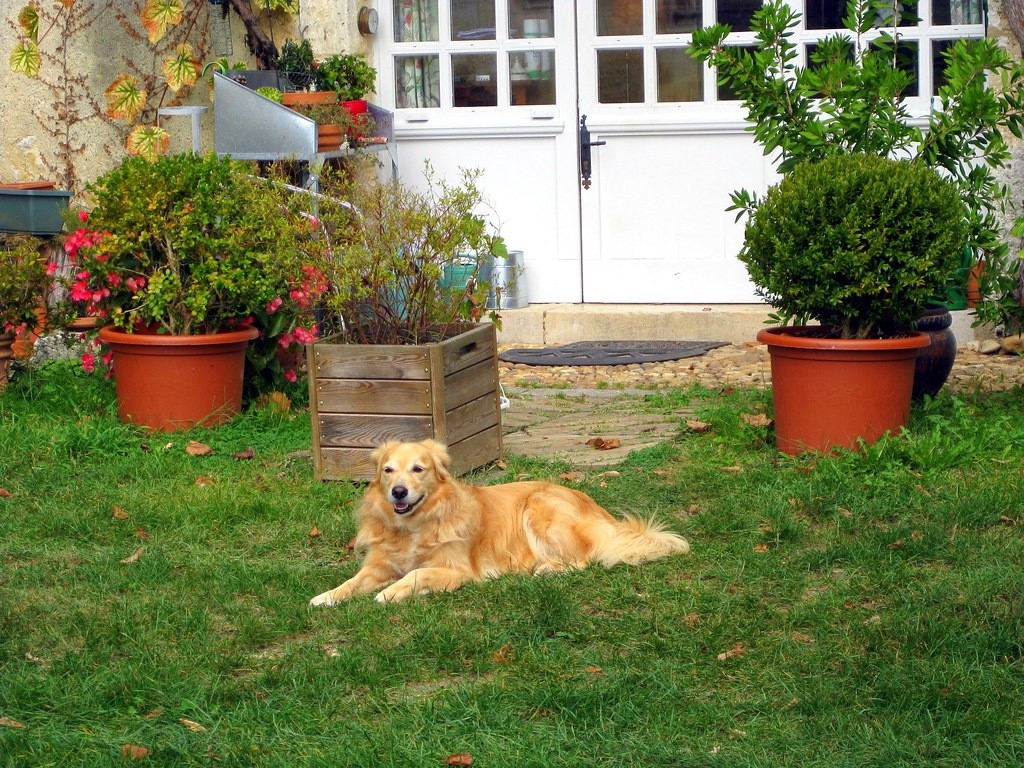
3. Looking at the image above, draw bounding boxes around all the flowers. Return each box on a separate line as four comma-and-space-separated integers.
60, 149, 329, 383
0, 233, 58, 335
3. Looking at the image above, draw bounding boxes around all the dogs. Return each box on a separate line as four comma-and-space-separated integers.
309, 438, 690, 606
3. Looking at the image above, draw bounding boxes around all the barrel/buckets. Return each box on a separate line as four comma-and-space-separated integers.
478, 252, 530, 310
444, 254, 478, 309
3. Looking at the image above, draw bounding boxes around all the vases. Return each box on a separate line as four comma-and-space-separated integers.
0, 333, 17, 393
966, 259, 988, 308
97, 323, 259, 433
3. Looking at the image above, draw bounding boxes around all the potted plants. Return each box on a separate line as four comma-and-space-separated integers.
304, 151, 523, 483
738, 152, 962, 465
271, 37, 336, 110
316, 49, 378, 146
685, 0, 1024, 407
292, 100, 377, 152
